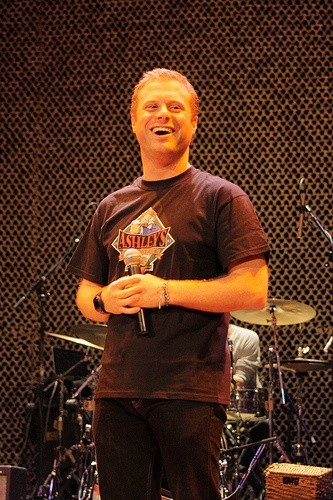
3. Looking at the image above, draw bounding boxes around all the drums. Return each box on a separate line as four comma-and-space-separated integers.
28, 379, 94, 462
225, 386, 282, 420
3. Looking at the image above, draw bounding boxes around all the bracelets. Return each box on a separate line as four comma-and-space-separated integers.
162, 281, 168, 307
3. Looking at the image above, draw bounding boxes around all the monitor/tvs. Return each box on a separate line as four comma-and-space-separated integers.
52, 347, 87, 379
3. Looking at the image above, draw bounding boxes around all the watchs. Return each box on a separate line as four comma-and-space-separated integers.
93, 291, 108, 314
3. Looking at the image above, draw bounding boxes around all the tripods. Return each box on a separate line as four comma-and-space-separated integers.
35, 378, 91, 500
232, 310, 294, 500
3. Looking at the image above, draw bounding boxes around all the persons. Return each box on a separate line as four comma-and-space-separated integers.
64, 67, 271, 500
227, 324, 263, 412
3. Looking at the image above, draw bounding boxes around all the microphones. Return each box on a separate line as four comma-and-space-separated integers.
124, 248, 149, 336
297, 184, 307, 239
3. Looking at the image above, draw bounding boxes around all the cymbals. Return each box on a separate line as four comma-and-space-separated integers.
229, 298, 318, 325
72, 324, 107, 347
44, 330, 104, 351
264, 357, 332, 373
